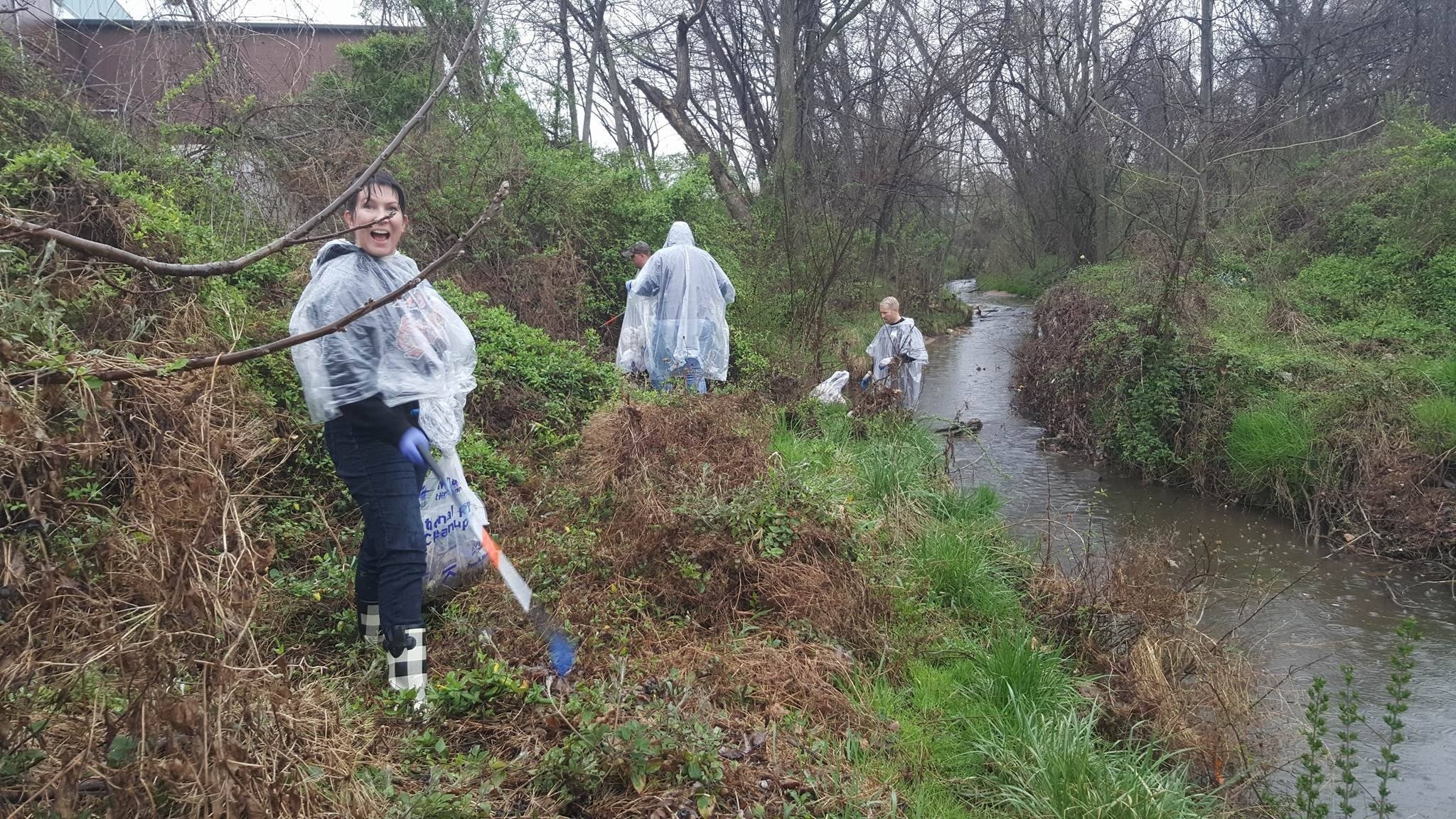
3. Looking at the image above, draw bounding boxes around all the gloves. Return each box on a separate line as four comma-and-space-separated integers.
861, 370, 873, 390
879, 357, 892, 371
398, 425, 430, 466
625, 279, 633, 291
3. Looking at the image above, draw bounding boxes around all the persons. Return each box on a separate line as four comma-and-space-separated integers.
626, 221, 735, 396
861, 296, 929, 411
622, 241, 651, 269
289, 169, 476, 711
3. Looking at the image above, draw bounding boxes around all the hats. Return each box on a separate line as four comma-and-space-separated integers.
622, 240, 650, 257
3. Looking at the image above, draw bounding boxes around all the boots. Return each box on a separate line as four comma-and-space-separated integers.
385, 629, 449, 724
357, 597, 385, 654
